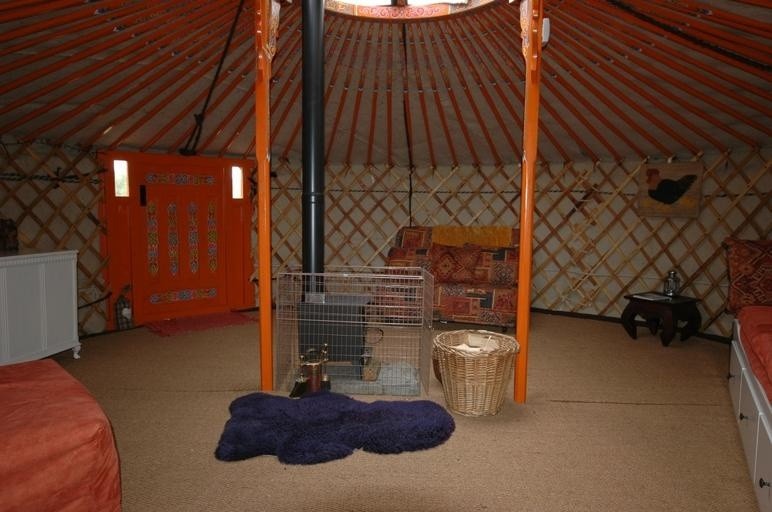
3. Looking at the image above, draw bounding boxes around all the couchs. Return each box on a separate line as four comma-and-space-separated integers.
374, 224, 520, 329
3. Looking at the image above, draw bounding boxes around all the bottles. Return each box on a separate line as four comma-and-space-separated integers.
664, 270, 681, 296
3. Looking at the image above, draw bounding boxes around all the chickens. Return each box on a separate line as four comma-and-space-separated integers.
643, 165, 698, 211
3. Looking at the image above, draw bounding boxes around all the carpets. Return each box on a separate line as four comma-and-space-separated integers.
144, 311, 260, 337
215, 393, 456, 463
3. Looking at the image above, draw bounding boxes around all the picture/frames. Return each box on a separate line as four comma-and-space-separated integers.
638, 161, 702, 219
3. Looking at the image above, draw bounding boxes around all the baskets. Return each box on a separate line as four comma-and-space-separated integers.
432, 329, 520, 418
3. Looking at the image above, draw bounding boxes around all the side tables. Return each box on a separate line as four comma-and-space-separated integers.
621, 292, 702, 346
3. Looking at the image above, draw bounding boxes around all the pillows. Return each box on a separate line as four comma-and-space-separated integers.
463, 241, 519, 287
724, 236, 772, 311
431, 240, 481, 286
387, 244, 430, 278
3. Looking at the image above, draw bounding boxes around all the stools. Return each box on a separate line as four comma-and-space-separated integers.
0, 359, 120, 512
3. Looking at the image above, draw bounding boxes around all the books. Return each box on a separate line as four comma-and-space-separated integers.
634, 291, 673, 304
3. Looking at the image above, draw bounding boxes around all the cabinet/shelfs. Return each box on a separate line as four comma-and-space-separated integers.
0, 246, 82, 365
726, 320, 772, 512
